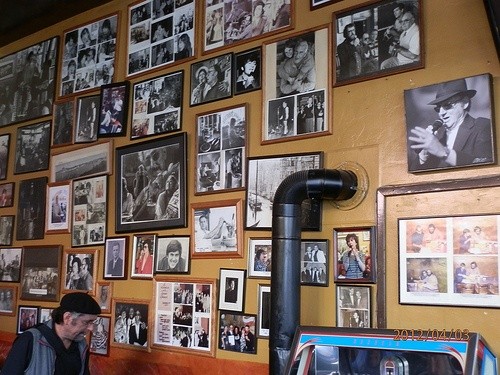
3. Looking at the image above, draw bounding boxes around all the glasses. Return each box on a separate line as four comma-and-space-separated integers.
398, 17, 414, 24
433, 99, 463, 113
71, 316, 98, 326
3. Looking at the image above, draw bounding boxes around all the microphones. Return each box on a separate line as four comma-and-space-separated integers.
430, 119, 444, 130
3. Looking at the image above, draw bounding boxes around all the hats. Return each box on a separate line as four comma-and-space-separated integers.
196, 66, 209, 80
427, 79, 477, 106
60, 293, 102, 315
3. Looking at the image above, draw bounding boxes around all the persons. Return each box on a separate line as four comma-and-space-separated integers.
0, 292, 101, 375
0, 0, 494, 352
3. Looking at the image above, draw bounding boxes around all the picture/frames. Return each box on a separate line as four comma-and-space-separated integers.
114, 131, 188, 233
231, 45, 263, 96
301, 238, 330, 287
398, 213, 500, 309
333, 226, 377, 284
257, 283, 271, 338
218, 310, 257, 354
189, 198, 244, 259
217, 268, 247, 313
261, 22, 333, 145
201, 0, 296, 56
336, 285, 372, 329
244, 151, 324, 232
154, 234, 191, 274
150, 276, 219, 359
0, 9, 157, 357
125, 0, 200, 81
195, 102, 248, 196
129, 69, 184, 140
332, 0, 425, 88
247, 237, 272, 280
190, 52, 234, 107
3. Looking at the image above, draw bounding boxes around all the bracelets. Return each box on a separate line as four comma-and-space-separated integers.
442, 148, 450, 161
